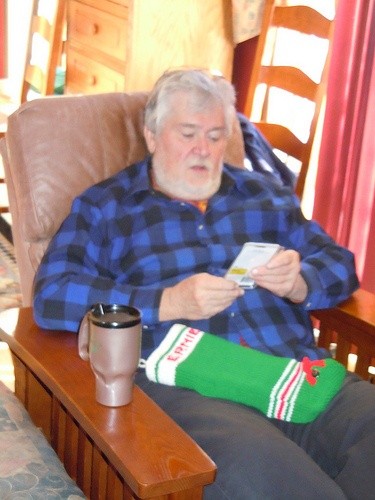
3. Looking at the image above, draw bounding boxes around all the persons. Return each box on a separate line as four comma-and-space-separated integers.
31, 69, 375, 500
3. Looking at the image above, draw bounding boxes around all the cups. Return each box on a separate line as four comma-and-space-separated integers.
77, 303, 142, 407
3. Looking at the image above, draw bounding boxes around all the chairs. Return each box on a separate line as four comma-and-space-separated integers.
1, 91, 375, 499
